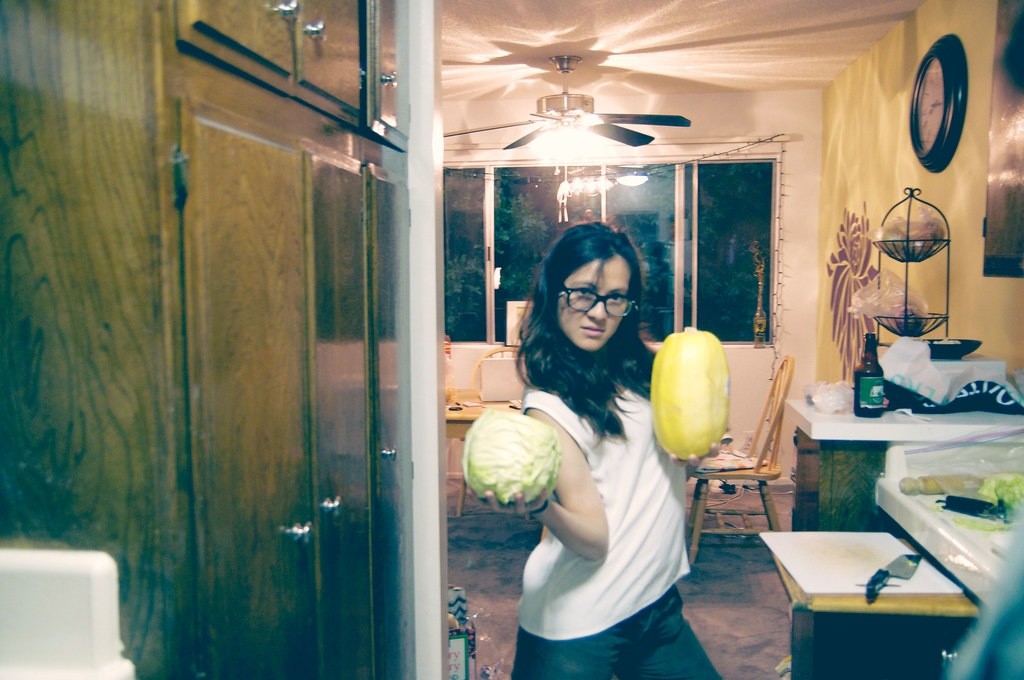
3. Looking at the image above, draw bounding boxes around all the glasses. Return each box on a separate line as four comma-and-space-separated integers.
564, 283, 636, 318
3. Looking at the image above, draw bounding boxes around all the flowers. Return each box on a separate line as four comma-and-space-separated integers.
748, 239, 766, 303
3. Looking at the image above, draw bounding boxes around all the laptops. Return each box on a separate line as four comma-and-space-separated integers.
478, 358, 527, 402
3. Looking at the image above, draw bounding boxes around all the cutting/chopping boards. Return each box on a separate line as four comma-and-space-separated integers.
758, 532, 963, 594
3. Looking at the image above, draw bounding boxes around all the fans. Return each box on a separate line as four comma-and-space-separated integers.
445, 54, 690, 150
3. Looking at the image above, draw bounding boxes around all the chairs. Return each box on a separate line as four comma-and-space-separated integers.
456, 346, 525, 518
687, 355, 794, 561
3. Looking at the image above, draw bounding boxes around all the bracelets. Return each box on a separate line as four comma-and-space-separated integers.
525, 499, 550, 514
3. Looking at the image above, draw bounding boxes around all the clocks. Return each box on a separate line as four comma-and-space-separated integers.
909, 33, 968, 172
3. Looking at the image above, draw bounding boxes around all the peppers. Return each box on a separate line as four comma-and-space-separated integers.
934, 495, 995, 518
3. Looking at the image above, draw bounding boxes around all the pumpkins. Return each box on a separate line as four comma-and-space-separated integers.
651, 331, 730, 460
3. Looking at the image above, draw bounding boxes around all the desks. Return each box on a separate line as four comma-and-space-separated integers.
444, 388, 524, 440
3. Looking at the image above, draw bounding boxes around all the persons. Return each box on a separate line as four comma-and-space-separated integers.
463, 221, 733, 680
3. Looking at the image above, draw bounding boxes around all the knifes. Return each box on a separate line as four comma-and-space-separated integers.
866, 555, 921, 597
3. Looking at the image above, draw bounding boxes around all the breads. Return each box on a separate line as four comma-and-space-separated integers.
876, 220, 946, 260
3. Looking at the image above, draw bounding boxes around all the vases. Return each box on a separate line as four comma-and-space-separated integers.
753, 303, 767, 348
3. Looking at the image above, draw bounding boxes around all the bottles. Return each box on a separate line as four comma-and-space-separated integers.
854, 333, 884, 417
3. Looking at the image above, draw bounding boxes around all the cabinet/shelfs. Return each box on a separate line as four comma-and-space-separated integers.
783, 395, 1024, 537
0, 0, 417, 679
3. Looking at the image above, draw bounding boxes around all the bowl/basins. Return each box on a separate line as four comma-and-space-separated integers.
922, 339, 982, 359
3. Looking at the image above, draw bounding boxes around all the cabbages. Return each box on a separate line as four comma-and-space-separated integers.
977, 472, 1024, 508
463, 409, 560, 502
